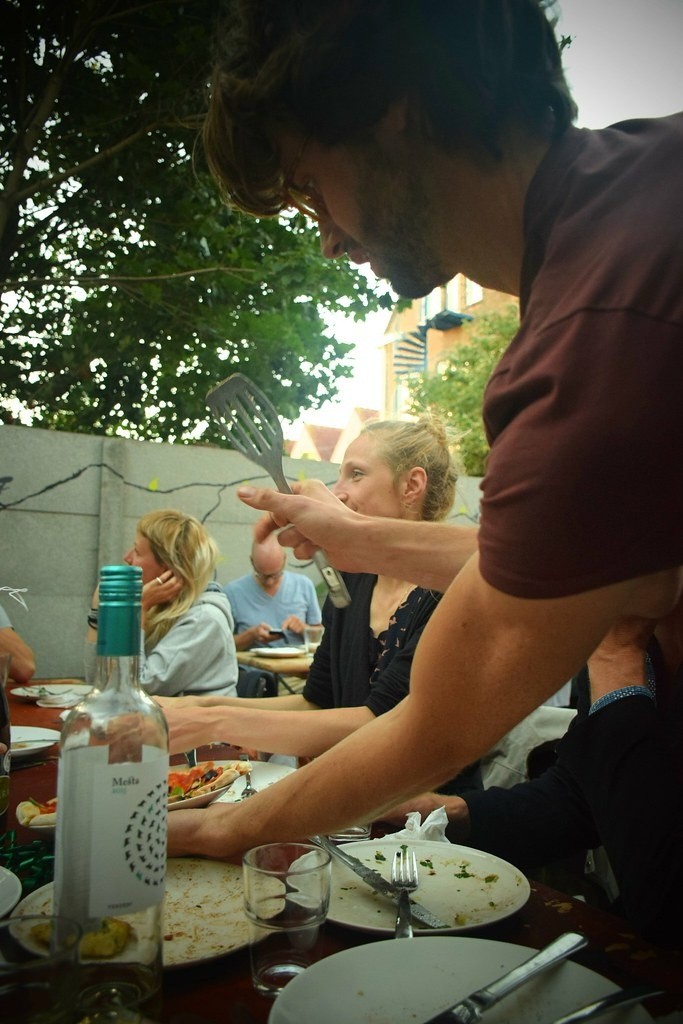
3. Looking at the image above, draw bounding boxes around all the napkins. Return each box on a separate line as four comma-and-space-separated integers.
36, 694, 82, 709
374, 805, 454, 844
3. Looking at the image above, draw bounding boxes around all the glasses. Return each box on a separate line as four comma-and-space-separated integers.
280, 138, 328, 222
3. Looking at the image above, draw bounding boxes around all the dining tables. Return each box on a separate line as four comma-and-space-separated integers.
0, 676, 683, 1024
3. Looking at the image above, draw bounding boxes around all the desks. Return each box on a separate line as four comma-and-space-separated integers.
237, 651, 310, 693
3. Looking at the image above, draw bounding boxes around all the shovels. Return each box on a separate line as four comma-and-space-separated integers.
207, 371, 351, 610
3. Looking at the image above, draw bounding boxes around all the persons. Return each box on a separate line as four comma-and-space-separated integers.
225, 533, 325, 698
83, 507, 238, 699
168, 1, 683, 1024
98, 409, 484, 835
0, 603, 36, 684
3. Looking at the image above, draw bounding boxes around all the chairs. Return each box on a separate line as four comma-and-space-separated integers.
234, 666, 278, 762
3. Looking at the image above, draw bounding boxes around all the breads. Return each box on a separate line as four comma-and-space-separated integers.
15, 762, 252, 826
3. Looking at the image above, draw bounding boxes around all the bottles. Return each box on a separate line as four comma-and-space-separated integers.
52, 564, 171, 1010
0, 672, 17, 867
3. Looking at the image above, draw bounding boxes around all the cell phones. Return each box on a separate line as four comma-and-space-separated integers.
269, 630, 286, 638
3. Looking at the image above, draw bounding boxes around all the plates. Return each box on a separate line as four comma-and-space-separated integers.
166, 759, 301, 805
250, 648, 305, 658
0, 864, 21, 919
36, 699, 79, 708
28, 782, 235, 837
269, 936, 652, 1024
10, 725, 62, 756
10, 856, 287, 970
10, 684, 93, 701
286, 838, 531, 933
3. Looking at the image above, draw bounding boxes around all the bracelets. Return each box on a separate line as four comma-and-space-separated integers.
87, 608, 97, 631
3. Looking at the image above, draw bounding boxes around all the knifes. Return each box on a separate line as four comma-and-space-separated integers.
308, 834, 451, 929
421, 931, 589, 1024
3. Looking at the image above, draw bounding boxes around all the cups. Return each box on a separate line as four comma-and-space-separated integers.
0, 651, 12, 691
0, 914, 85, 1024
241, 841, 331, 1000
303, 627, 323, 657
329, 822, 371, 841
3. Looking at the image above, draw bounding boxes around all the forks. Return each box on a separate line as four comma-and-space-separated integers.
239, 749, 258, 801
391, 850, 419, 940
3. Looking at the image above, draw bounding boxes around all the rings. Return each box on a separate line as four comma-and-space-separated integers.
156, 577, 163, 585
